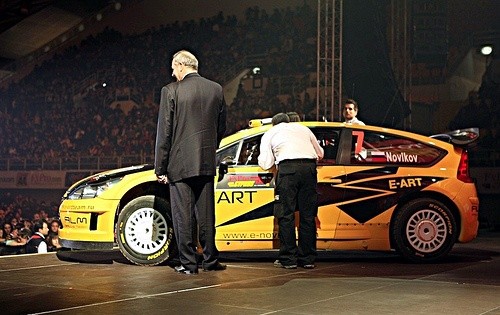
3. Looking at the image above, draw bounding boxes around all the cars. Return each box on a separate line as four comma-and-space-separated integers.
56, 117, 481, 268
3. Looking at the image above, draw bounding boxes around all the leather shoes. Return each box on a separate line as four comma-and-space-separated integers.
174, 264, 198, 274
204, 262, 226, 272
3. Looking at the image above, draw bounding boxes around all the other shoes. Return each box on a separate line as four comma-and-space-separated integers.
274, 259, 297, 268
299, 263, 315, 268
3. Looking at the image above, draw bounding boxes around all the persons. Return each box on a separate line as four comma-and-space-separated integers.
0, 0, 500, 256
155, 50, 228, 275
258, 112, 326, 268
